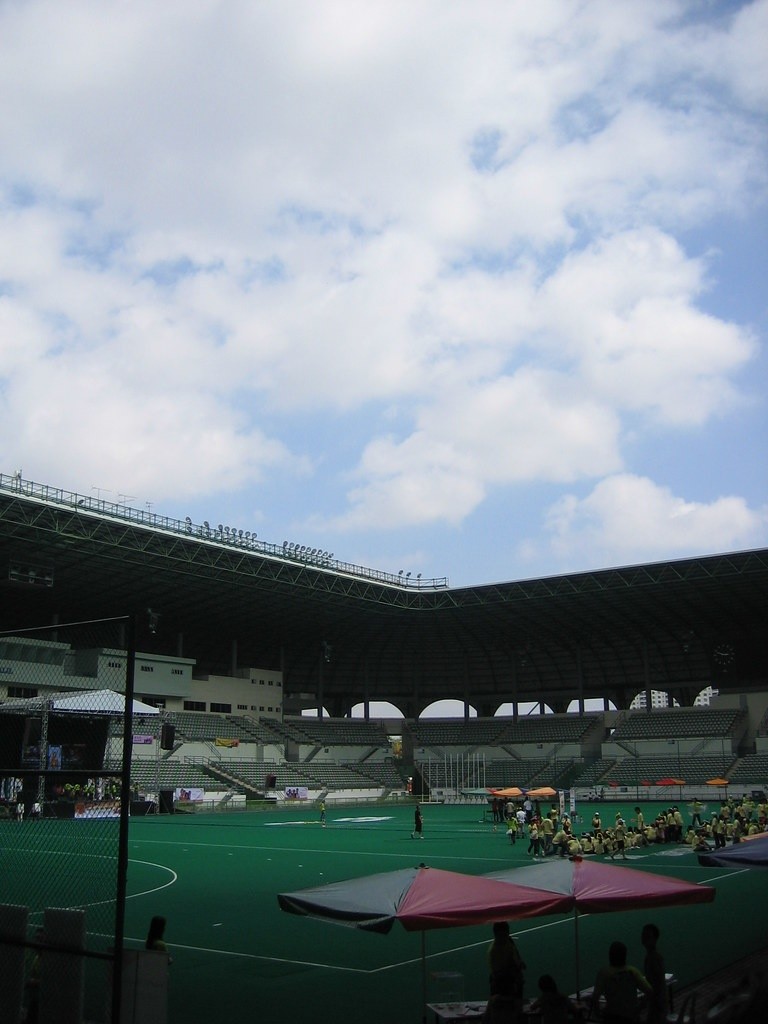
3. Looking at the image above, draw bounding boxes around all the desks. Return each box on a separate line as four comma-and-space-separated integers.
426, 999, 545, 1024
568, 977, 674, 1024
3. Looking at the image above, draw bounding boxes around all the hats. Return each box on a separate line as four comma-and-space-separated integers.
606, 831, 610, 835
618, 818, 625, 823
586, 833, 590, 837
594, 813, 599, 816
517, 807, 522, 809
597, 833, 602, 838
711, 811, 716, 815
688, 825, 694, 828
615, 812, 621, 819
581, 832, 586, 837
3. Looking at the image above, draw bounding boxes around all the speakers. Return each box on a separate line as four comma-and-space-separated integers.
161, 725, 175, 750
130, 801, 156, 816
160, 791, 173, 813
265, 776, 276, 788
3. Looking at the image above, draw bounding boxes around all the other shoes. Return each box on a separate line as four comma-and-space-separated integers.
645, 843, 649, 846
527, 852, 530, 855
611, 855, 614, 860
622, 858, 628, 860
743, 793, 747, 796
635, 845, 640, 848
535, 854, 538, 856
541, 851, 545, 856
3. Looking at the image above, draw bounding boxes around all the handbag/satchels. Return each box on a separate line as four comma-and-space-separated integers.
506, 829, 512, 834
594, 820, 600, 828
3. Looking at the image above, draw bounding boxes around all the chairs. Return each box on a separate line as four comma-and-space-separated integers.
666, 993, 696, 1024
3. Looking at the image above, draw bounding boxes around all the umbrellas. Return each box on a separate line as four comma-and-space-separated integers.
473, 855, 716, 993
655, 778, 685, 785
278, 836, 576, 1024
461, 787, 571, 797
706, 778, 728, 785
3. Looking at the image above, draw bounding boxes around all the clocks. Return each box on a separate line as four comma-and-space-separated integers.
713, 644, 735, 666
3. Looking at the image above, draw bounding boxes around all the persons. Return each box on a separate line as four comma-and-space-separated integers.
411, 806, 424, 840
320, 800, 327, 821
483, 921, 668, 1024
589, 788, 605, 802
16, 781, 139, 822
492, 797, 768, 861
